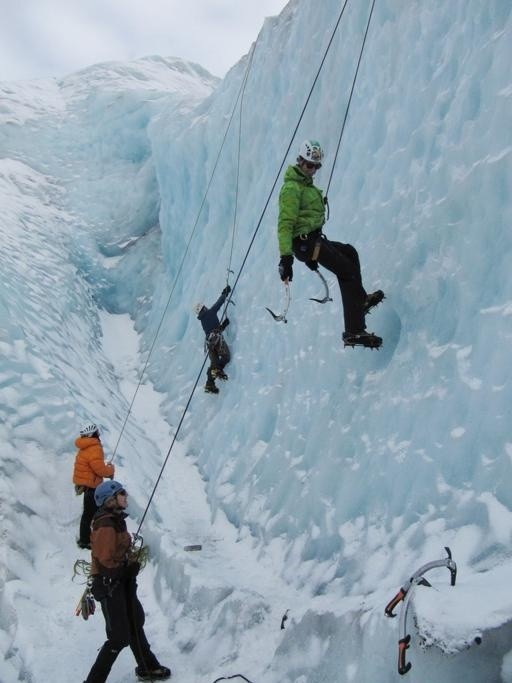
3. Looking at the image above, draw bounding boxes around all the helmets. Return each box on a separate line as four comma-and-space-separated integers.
192, 302, 205, 314
94, 480, 122, 507
79, 422, 98, 438
299, 138, 324, 162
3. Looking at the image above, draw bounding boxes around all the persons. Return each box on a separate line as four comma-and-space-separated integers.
192, 284, 232, 394
278, 140, 385, 350
72, 423, 115, 550
76, 479, 171, 682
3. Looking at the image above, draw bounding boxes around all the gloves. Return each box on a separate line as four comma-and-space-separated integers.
221, 285, 231, 297
305, 256, 319, 271
222, 318, 229, 329
278, 255, 294, 282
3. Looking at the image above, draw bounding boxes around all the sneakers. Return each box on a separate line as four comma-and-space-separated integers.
362, 290, 384, 315
135, 667, 171, 681
342, 330, 383, 348
205, 382, 219, 394
211, 368, 229, 380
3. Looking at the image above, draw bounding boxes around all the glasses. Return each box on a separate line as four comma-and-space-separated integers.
305, 163, 322, 169
115, 489, 127, 496
